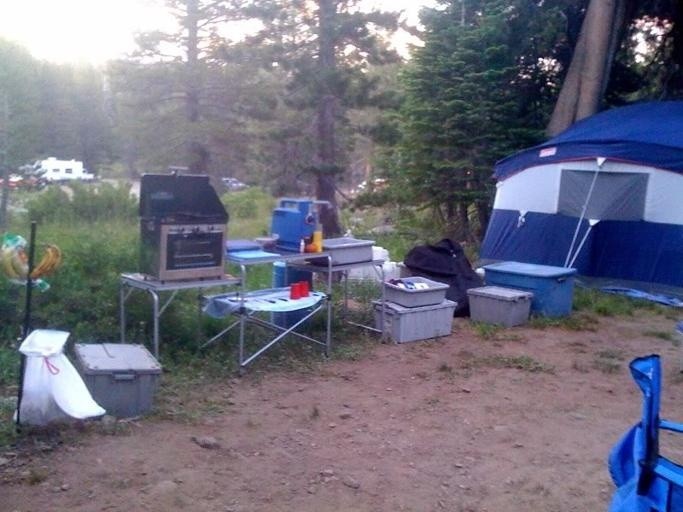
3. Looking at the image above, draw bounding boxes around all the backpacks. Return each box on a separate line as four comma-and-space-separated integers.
403, 237, 481, 318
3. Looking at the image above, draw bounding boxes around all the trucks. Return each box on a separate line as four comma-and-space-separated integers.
34, 156, 94, 182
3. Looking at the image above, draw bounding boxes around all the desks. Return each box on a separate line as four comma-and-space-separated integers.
280, 259, 388, 354
115, 273, 243, 363
196, 239, 331, 367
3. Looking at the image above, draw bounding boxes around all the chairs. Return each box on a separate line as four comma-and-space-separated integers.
608, 354, 683, 512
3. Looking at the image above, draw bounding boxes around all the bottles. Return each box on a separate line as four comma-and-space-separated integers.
299, 237, 304, 253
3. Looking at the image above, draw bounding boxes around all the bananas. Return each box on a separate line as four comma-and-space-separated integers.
29, 244, 61, 280
4, 249, 23, 279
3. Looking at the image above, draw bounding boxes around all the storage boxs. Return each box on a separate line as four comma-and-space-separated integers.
370, 298, 458, 343
383, 276, 451, 307
73, 342, 162, 415
483, 261, 576, 319
466, 285, 533, 328
310, 237, 376, 265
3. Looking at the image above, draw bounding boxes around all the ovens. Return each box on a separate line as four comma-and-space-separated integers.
138, 215, 225, 284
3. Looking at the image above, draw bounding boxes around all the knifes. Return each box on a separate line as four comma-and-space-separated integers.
256, 297, 276, 304
271, 297, 287, 302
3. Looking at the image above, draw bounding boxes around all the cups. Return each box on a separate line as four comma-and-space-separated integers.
289, 282, 301, 300
300, 280, 309, 298
311, 223, 323, 252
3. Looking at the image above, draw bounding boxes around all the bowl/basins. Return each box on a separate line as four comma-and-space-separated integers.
255, 238, 276, 252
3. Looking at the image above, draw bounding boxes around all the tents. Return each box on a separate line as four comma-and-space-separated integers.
480, 101, 682, 309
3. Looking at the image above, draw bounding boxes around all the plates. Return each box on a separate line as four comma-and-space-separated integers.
227, 296, 248, 303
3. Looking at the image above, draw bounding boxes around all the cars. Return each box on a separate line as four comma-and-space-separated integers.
221, 176, 244, 193
0, 171, 46, 195
